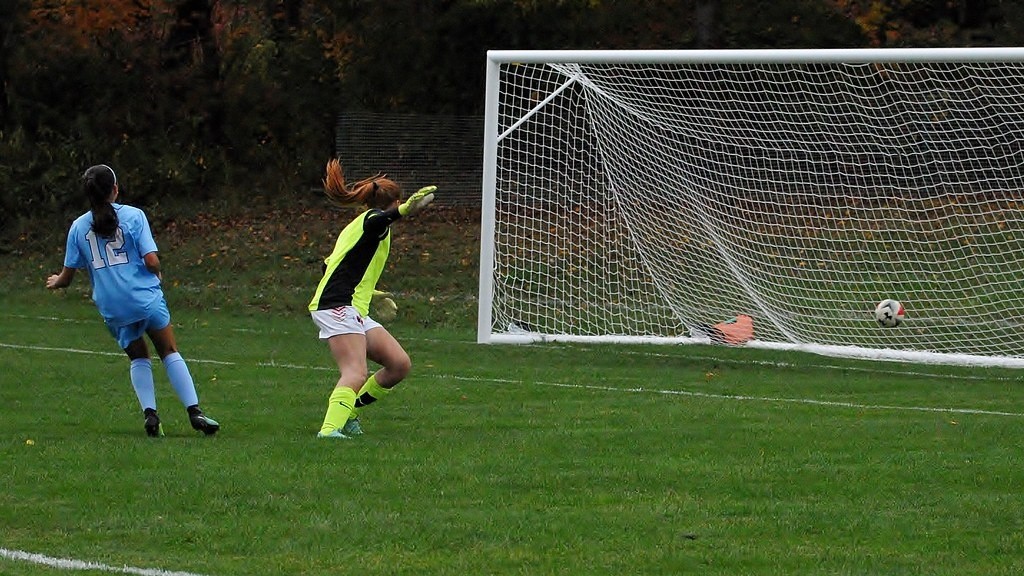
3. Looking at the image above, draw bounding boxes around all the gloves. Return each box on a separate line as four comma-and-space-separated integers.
398, 186, 437, 216
370, 290, 398, 322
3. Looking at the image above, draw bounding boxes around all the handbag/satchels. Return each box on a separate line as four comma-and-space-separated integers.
712, 314, 754, 345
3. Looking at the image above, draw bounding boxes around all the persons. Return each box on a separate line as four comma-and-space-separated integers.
309, 158, 435, 439
47, 165, 217, 437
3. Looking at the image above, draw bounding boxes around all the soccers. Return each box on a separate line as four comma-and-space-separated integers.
874, 299, 905, 329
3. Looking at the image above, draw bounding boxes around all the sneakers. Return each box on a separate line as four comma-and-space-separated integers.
343, 416, 363, 434
190, 414, 219, 435
144, 415, 165, 438
317, 431, 352, 439
